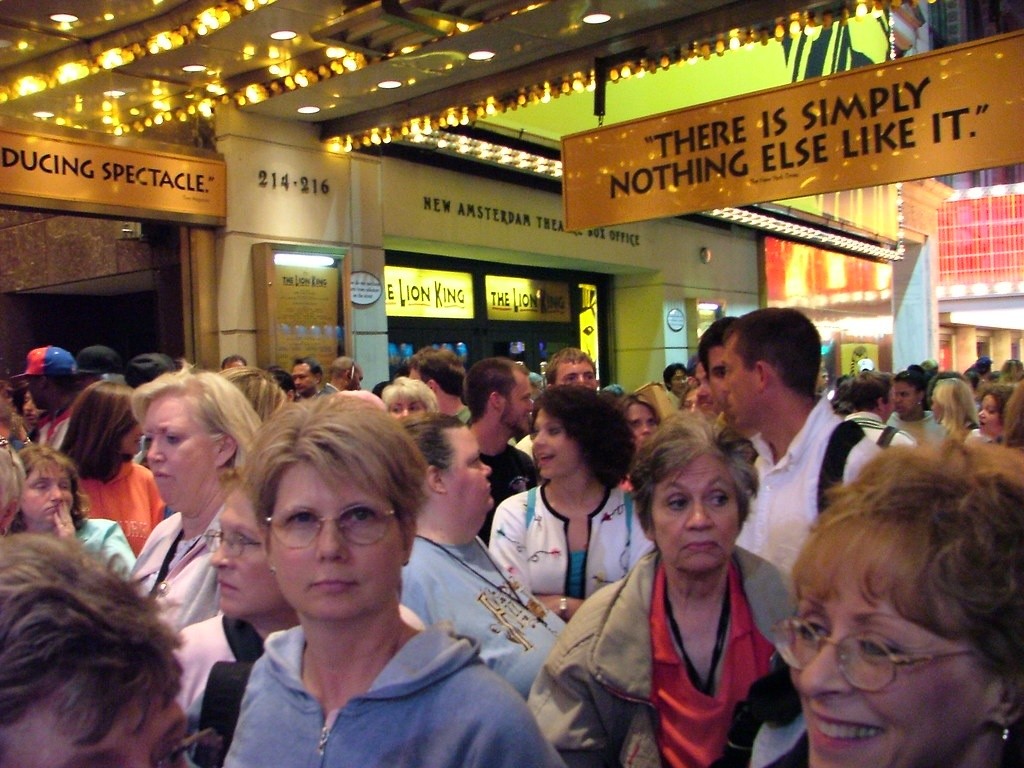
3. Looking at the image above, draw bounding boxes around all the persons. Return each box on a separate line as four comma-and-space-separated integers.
0, 306, 1024, 768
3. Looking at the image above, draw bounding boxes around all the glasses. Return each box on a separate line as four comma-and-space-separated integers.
265, 505, 393, 549
203, 529, 262, 555
771, 616, 973, 691
156, 728, 223, 768
892, 371, 914, 379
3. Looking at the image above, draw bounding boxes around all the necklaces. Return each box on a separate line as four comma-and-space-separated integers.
416, 534, 546, 625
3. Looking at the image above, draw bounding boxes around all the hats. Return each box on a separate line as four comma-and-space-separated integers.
74, 346, 119, 374
858, 359, 875, 372
10, 346, 77, 380
126, 352, 174, 387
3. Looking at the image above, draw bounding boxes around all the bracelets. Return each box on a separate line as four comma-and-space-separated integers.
559, 595, 568, 617
